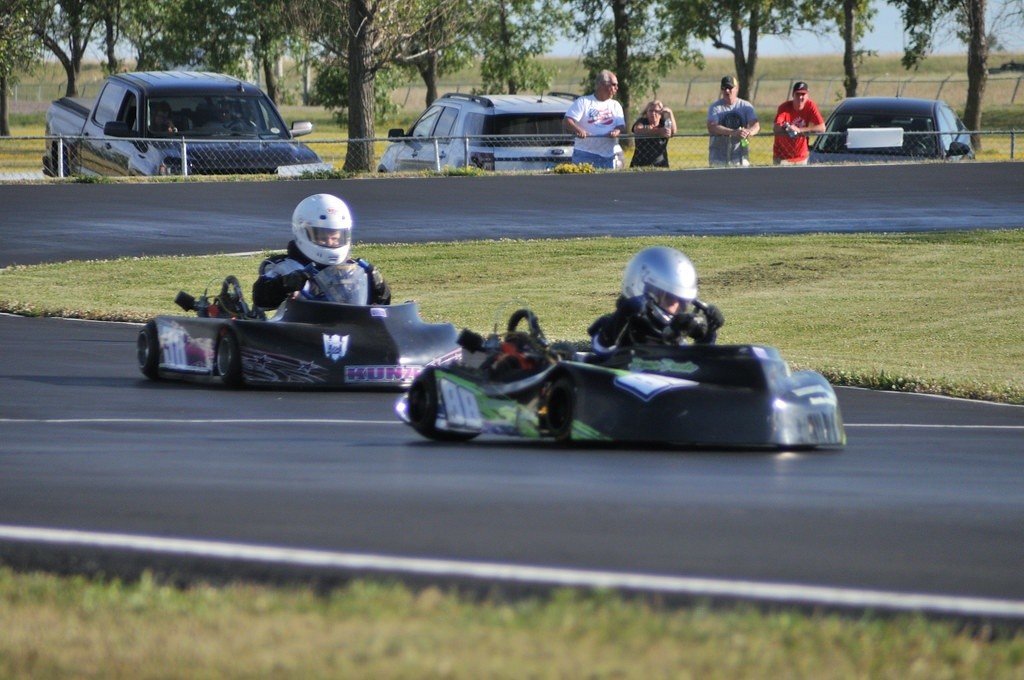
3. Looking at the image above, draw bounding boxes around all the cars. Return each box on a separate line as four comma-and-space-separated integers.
805, 92, 978, 163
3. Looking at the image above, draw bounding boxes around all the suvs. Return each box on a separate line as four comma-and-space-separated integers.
373, 91, 625, 176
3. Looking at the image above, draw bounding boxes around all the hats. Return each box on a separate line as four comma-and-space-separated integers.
720, 76, 737, 89
793, 82, 809, 94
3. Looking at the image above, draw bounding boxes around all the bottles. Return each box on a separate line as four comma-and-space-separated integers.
664, 116, 671, 129
782, 121, 797, 137
739, 126, 749, 147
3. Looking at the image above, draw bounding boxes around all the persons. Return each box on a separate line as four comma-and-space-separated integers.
707, 75, 761, 166
252, 193, 391, 313
772, 81, 825, 165
629, 99, 677, 168
153, 99, 254, 133
586, 245, 727, 358
564, 69, 627, 169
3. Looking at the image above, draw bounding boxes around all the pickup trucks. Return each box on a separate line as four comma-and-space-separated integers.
41, 68, 337, 177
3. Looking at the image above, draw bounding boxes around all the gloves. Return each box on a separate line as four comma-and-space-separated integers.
368, 266, 384, 296
622, 295, 647, 322
707, 304, 724, 331
279, 270, 306, 296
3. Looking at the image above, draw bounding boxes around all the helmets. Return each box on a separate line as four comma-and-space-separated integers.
293, 194, 353, 267
619, 246, 698, 336
